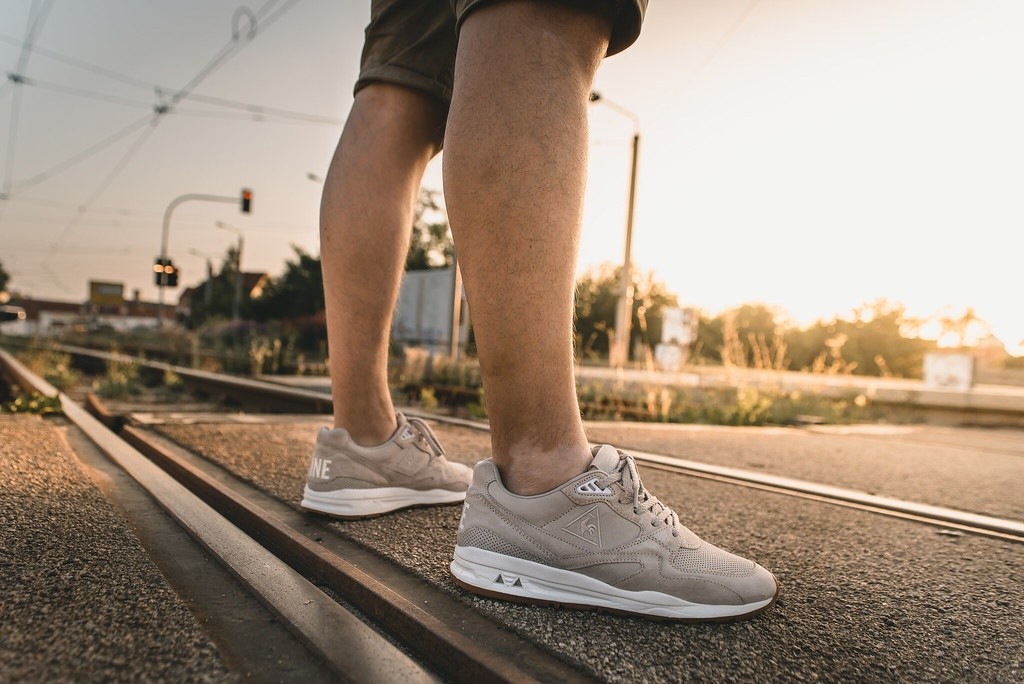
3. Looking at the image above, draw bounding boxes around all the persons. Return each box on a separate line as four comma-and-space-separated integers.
300, 1, 778, 620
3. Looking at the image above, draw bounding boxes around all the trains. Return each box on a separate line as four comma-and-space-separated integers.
0, 305, 27, 338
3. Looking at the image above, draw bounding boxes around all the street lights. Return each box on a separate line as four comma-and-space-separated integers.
216, 221, 244, 336
588, 91, 641, 368
189, 247, 214, 308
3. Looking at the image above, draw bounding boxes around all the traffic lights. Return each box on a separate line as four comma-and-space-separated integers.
241, 189, 251, 213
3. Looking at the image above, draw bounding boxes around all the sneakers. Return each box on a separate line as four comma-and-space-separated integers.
450, 444, 780, 624
300, 414, 472, 520
